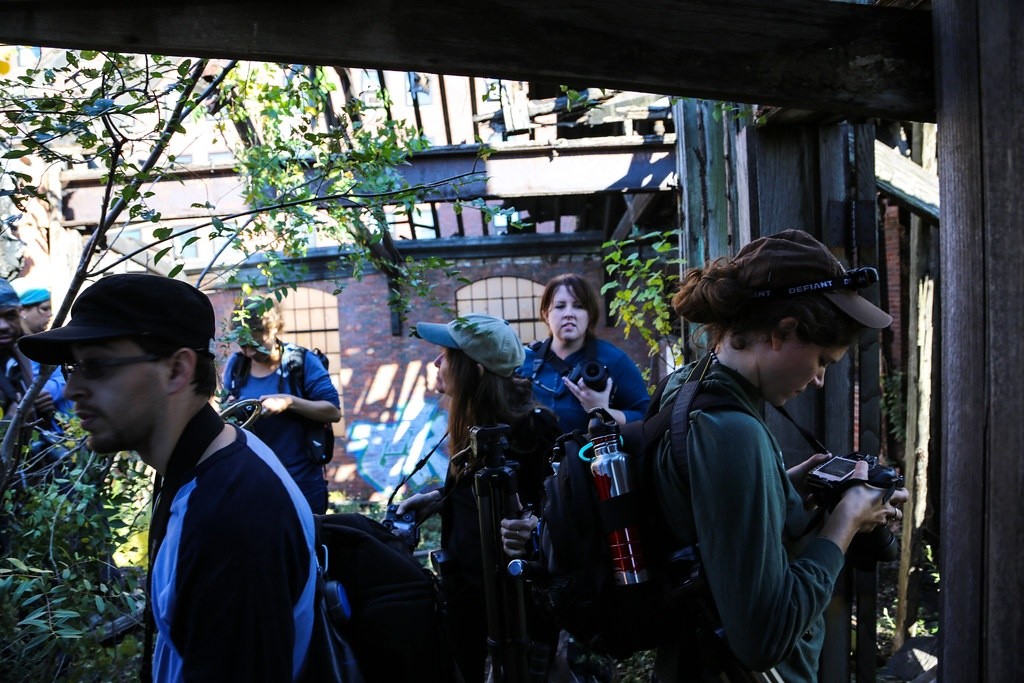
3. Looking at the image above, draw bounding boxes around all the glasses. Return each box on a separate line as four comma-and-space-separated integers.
60, 349, 182, 379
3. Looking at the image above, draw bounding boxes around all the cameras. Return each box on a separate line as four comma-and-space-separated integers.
806, 450, 898, 571
383, 505, 419, 555
583, 360, 617, 406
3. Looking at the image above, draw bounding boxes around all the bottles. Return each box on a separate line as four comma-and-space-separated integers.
588, 407, 653, 586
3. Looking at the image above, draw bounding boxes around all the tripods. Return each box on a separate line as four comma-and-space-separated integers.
450, 423, 533, 683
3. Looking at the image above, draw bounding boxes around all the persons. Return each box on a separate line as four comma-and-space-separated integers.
645, 230, 906, 683
394, 314, 571, 682
1, 275, 137, 612
17, 275, 316, 683
514, 274, 651, 432
220, 297, 341, 515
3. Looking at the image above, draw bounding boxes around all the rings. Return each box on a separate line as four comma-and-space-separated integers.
516, 531, 518, 536
890, 513, 896, 521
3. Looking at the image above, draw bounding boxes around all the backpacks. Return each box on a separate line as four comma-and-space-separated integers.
528, 363, 748, 683
288, 344, 334, 463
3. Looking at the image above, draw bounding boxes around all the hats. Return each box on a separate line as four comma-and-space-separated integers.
16, 275, 216, 366
0, 277, 20, 311
726, 228, 894, 329
417, 314, 526, 377
17, 289, 49, 306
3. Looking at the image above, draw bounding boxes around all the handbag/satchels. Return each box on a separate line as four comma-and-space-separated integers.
300, 504, 459, 683
